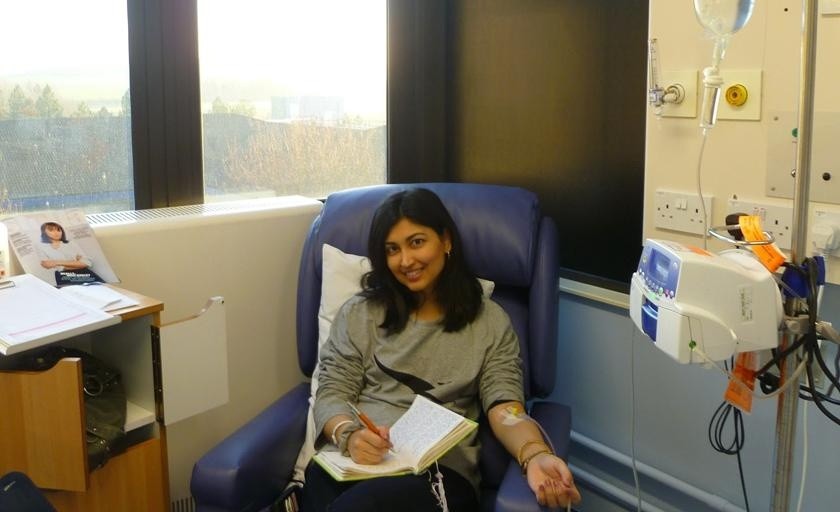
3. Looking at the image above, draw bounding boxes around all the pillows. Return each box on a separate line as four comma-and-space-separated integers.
292, 241, 497, 487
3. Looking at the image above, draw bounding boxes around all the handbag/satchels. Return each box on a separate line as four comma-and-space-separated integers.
25, 344, 130, 477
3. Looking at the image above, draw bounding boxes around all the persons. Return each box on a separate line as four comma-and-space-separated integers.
300, 185, 582, 510
34, 220, 104, 284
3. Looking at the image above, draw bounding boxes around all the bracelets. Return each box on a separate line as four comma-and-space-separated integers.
519, 449, 557, 480
516, 437, 550, 467
329, 417, 354, 447
337, 422, 362, 458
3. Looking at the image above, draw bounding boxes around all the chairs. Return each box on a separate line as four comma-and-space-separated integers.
189, 180, 574, 512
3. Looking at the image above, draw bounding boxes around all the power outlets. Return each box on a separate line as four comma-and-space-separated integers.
654, 188, 839, 260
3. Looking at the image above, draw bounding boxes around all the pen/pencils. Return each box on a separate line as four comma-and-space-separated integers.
346, 400, 397, 455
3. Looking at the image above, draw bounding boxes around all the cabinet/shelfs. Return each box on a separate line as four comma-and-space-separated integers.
0, 302, 174, 512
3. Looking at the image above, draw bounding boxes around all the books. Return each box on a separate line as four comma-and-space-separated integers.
0, 275, 123, 355
311, 392, 479, 483
5, 207, 121, 285
